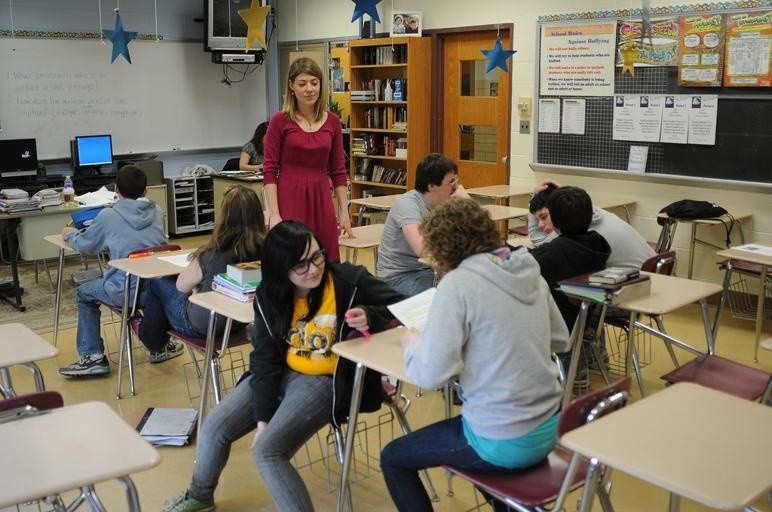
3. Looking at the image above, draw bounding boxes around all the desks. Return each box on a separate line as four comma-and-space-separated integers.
0, 400, 161, 511
349, 194, 402, 226
466, 184, 535, 199
418, 236, 532, 289
210, 171, 339, 225
656, 207, 753, 279
0, 322, 59, 506
592, 197, 637, 225
338, 223, 386, 274
333, 327, 460, 511
0, 200, 116, 313
709, 244, 772, 363
480, 204, 530, 225
188, 291, 256, 465
562, 268, 724, 406
43, 233, 170, 348
560, 382, 772, 511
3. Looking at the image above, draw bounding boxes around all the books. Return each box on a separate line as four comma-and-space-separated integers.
353, 158, 407, 185
362, 189, 385, 226
350, 79, 386, 101
37, 188, 59, 200
360, 45, 407, 65
363, 105, 407, 129
588, 267, 640, 284
351, 133, 398, 157
211, 260, 261, 303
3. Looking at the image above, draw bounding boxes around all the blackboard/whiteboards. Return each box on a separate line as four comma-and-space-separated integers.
531, 7, 772, 194
1, 29, 270, 161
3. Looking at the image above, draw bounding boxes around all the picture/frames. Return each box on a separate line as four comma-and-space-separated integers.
390, 11, 423, 37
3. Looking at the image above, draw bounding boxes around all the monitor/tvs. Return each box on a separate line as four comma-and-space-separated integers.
207, 0, 266, 50
0, 138, 38, 177
75, 134, 114, 179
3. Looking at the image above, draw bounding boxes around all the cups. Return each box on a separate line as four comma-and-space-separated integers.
63, 188, 74, 208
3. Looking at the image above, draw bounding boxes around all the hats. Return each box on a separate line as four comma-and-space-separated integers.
410, 19, 416, 24
395, 15, 403, 23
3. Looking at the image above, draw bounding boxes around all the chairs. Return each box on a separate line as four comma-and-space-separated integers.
441, 376, 632, 512
276, 318, 440, 512
715, 259, 772, 324
166, 318, 252, 410
646, 219, 681, 276
584, 250, 680, 398
0, 391, 106, 512
659, 355, 772, 405
508, 225, 527, 238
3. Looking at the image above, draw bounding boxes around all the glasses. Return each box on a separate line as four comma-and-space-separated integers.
441, 176, 459, 188
287, 248, 323, 276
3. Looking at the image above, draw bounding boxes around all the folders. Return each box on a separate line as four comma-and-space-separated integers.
134, 406, 197, 444
555, 271, 651, 306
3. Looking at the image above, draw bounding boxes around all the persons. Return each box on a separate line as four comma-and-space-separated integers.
60, 165, 184, 379
160, 220, 406, 512
402, 16, 413, 27
379, 198, 569, 512
405, 20, 418, 33
239, 121, 270, 172
505, 186, 611, 357
528, 179, 657, 390
377, 154, 474, 406
393, 15, 405, 33
264, 58, 357, 263
128, 185, 266, 350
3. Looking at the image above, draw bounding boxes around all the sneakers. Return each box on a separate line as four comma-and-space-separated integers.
148, 340, 185, 362
162, 489, 214, 512
59, 355, 109, 376
581, 347, 610, 369
563, 362, 590, 389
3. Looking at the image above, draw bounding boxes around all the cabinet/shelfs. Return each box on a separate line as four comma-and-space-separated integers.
165, 176, 214, 236
350, 37, 432, 226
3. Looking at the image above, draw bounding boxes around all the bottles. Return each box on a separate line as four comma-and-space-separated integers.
63, 176, 74, 204
